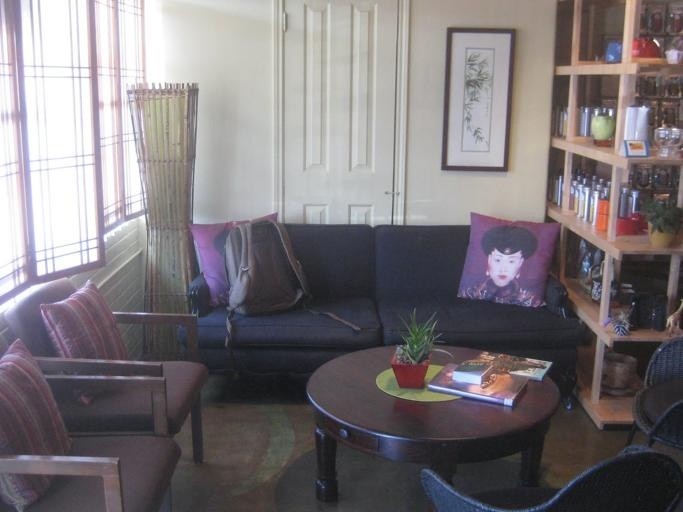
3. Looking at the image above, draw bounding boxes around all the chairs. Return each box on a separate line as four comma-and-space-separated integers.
1, 375, 182, 512
624, 336, 683, 449
0, 278, 209, 462
420, 445, 683, 512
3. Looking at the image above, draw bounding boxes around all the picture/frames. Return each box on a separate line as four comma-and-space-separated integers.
442, 27, 516, 172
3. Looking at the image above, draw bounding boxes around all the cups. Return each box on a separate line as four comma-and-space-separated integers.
654, 128, 683, 160
600, 352, 635, 396
664, 49, 681, 65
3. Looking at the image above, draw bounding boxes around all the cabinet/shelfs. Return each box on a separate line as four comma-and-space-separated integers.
546, 0, 683, 430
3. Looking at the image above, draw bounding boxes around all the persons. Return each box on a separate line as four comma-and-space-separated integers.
458, 225, 547, 309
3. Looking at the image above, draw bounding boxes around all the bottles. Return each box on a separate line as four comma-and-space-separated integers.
551, 105, 612, 140
547, 166, 640, 225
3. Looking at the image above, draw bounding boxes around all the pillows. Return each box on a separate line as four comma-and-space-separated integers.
0, 339, 75, 512
188, 213, 278, 307
457, 212, 562, 308
40, 280, 129, 404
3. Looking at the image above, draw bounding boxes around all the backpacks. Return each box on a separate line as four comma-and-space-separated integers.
223, 218, 312, 317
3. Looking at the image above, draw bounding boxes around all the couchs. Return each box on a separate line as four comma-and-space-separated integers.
178, 225, 578, 411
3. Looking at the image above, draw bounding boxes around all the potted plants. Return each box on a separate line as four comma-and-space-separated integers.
638, 196, 683, 248
390, 308, 454, 388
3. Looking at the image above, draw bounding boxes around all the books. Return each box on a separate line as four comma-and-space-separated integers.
427, 362, 529, 407
474, 351, 553, 382
